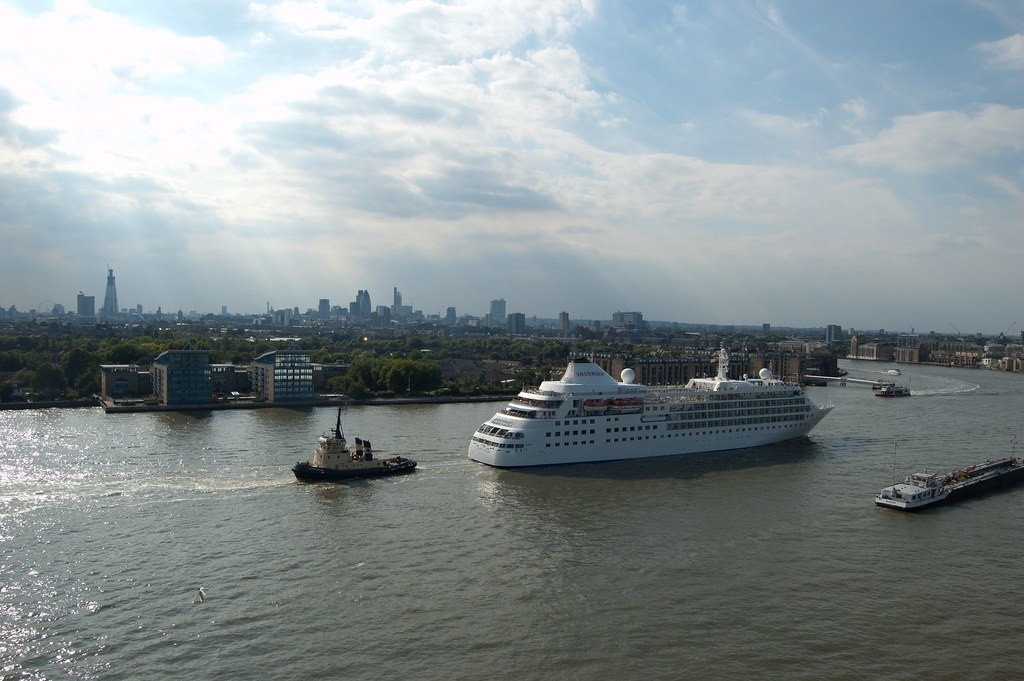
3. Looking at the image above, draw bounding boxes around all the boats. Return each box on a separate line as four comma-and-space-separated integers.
875, 385, 910, 396
291, 403, 417, 483
872, 381, 894, 389
875, 436, 1024, 512
888, 369, 901, 375
468, 342, 834, 468
